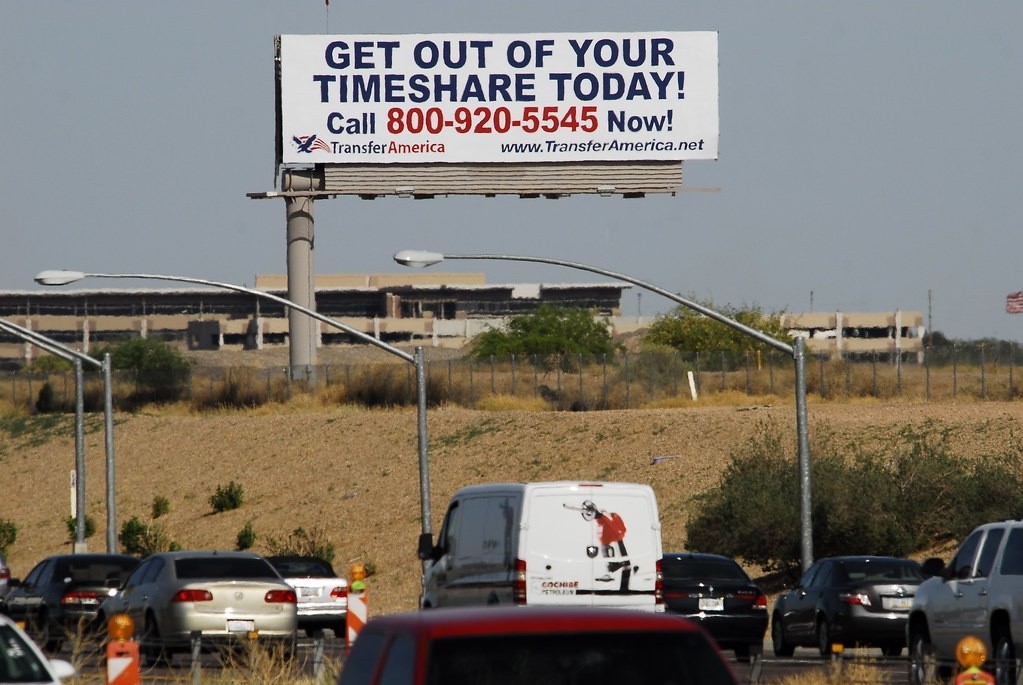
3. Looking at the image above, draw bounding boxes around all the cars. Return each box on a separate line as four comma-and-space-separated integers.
0, 550, 349, 685
338, 604, 736, 685
661, 520, 1023, 662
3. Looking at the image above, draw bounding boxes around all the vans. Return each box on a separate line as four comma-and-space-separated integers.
418, 479, 666, 614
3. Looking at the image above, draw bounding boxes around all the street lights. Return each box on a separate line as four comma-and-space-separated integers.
34, 269, 430, 574
391, 251, 812, 575
